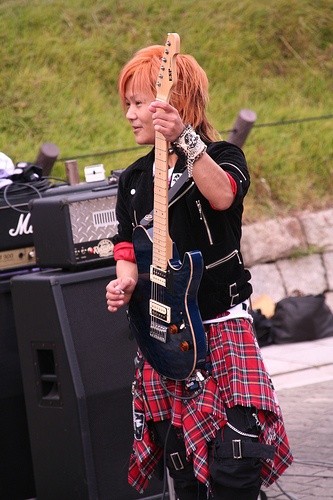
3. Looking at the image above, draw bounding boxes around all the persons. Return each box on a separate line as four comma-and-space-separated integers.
105, 46, 294, 500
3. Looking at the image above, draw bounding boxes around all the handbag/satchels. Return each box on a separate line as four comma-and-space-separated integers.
249, 309, 273, 348
272, 289, 333, 344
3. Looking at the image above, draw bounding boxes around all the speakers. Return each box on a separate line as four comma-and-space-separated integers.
0, 276, 35, 500
10, 265, 170, 500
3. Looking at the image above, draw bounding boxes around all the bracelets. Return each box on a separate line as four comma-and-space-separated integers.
171, 125, 207, 177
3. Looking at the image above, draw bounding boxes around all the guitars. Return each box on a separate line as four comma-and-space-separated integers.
127, 33, 208, 382
